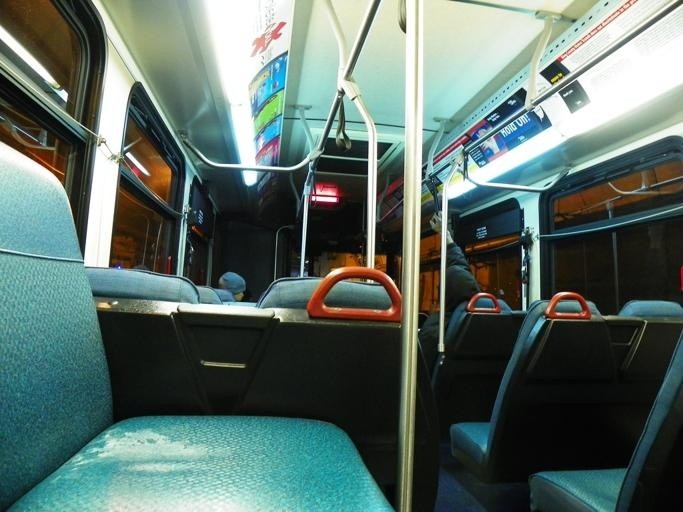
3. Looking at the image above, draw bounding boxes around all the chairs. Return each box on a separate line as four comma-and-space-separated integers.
1, 137, 399, 507
525, 332, 683, 507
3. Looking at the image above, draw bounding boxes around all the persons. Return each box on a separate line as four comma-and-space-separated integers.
218, 271, 246, 301
418, 209, 480, 379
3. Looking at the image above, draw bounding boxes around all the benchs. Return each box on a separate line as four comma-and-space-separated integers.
426, 292, 600, 430
83, 264, 401, 508
450, 291, 683, 512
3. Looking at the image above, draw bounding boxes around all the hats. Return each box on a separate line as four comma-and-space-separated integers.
219, 272, 247, 294
